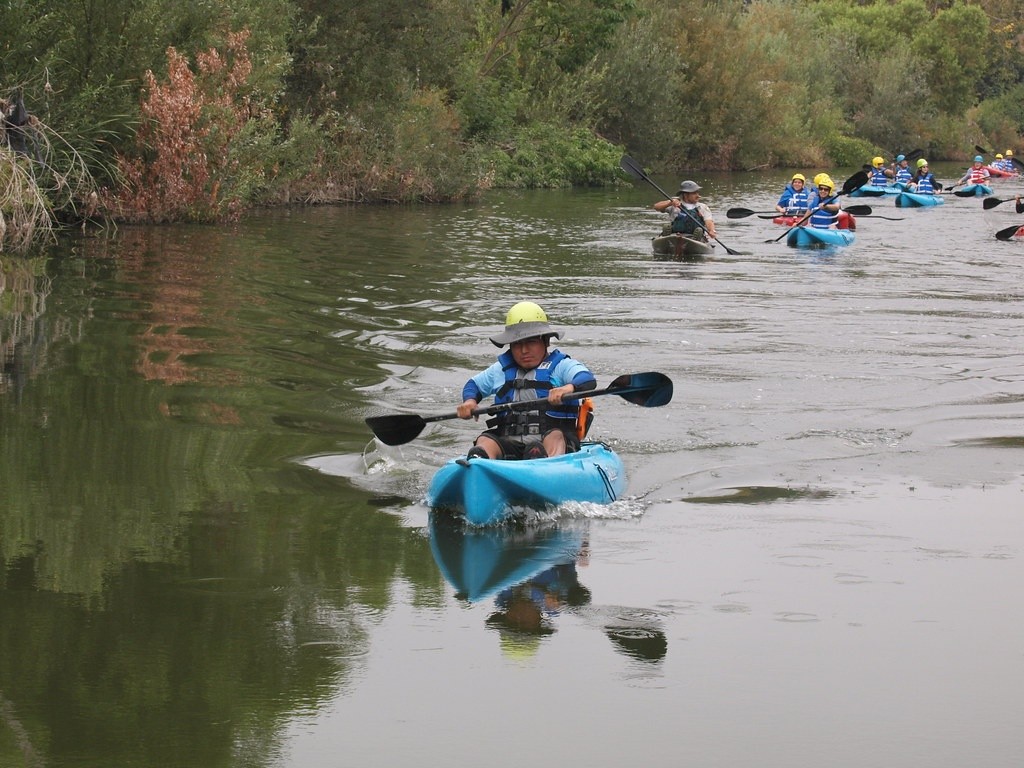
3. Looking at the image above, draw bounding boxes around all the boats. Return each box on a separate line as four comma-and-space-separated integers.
895, 192, 945, 208
982, 165, 1019, 178
650, 233, 716, 255
848, 182, 916, 198
426, 510, 587, 603
773, 213, 857, 230
953, 184, 994, 197
787, 224, 855, 250
428, 438, 625, 526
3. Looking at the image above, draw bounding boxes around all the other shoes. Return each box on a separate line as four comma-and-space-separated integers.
466, 447, 490, 460
692, 227, 703, 241
662, 224, 671, 237
523, 441, 548, 461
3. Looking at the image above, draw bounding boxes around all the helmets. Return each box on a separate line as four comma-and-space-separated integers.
897, 155, 905, 162
490, 302, 566, 348
791, 174, 806, 188
917, 159, 928, 168
814, 173, 829, 188
818, 178, 834, 196
1005, 150, 1013, 155
873, 157, 884, 168
676, 180, 704, 196
996, 154, 1003, 160
974, 155, 983, 162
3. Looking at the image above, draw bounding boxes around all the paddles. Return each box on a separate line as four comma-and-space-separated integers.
863, 190, 977, 198
944, 173, 1002, 191
995, 224, 1023, 241
962, 166, 1001, 179
982, 196, 1024, 210
975, 144, 996, 160
1010, 157, 1024, 169
872, 148, 925, 178
758, 215, 907, 221
362, 371, 674, 447
763, 170, 869, 243
726, 204, 872, 219
619, 153, 741, 255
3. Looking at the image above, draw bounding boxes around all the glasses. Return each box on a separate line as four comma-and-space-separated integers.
819, 187, 829, 191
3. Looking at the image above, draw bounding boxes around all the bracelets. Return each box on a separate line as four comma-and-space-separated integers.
712, 230, 716, 234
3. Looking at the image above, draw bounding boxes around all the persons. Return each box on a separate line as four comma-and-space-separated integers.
893, 155, 914, 184
906, 158, 941, 196
807, 173, 837, 206
958, 155, 990, 187
1005, 150, 1017, 173
793, 178, 840, 230
775, 174, 810, 214
989, 154, 1006, 171
1015, 195, 1024, 214
868, 157, 896, 186
456, 301, 597, 461
655, 181, 717, 242
